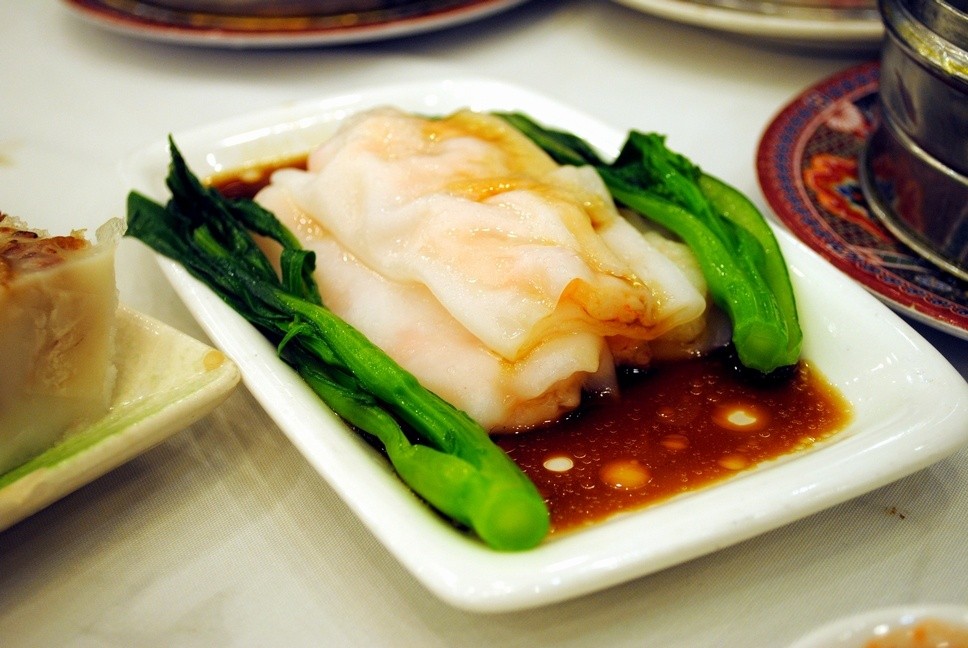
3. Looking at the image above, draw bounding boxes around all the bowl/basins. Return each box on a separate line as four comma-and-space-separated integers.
863, 0, 968, 285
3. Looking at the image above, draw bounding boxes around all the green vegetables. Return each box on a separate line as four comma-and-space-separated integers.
123, 132, 552, 551
489, 110, 804, 371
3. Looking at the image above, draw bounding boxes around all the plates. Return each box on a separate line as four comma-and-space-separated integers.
784, 605, 968, 648
0, 308, 240, 531
621, 0, 884, 50
751, 68, 968, 347
119, 74, 968, 613
51, 0, 522, 49
